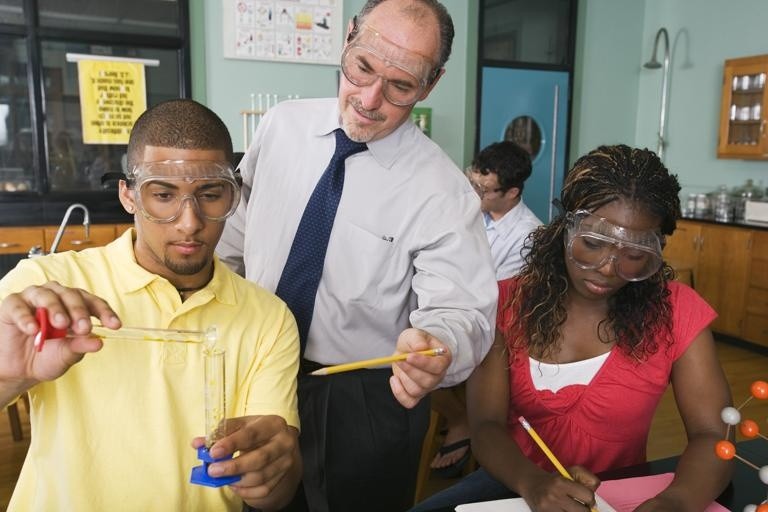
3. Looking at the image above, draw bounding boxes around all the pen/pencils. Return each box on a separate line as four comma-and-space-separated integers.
306, 348, 448, 375
517, 415, 600, 512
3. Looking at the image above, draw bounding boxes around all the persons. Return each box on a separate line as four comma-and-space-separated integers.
214, 0, 499, 512
467, 145, 736, 512
467, 141, 546, 282
0, 99, 303, 512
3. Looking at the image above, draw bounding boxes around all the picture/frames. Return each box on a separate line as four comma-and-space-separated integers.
221, 0, 345, 66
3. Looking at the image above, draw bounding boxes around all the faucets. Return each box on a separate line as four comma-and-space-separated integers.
50, 204, 89, 253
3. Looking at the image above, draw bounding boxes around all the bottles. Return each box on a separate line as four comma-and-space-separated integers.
684, 179, 762, 223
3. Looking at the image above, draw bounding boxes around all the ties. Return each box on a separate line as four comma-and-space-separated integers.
275, 128, 368, 361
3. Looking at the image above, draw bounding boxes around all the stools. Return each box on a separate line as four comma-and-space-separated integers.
411, 411, 478, 504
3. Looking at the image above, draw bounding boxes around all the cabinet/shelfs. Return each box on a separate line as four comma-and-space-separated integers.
718, 54, 768, 161
745, 229, 768, 348
662, 221, 754, 339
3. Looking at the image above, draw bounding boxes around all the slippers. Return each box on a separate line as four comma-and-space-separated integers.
429, 437, 471, 476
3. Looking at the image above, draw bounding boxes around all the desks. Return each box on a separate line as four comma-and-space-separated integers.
595, 438, 767, 512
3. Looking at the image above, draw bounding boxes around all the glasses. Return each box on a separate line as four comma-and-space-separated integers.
563, 208, 663, 283
340, 22, 434, 106
465, 165, 504, 199
131, 160, 243, 223
